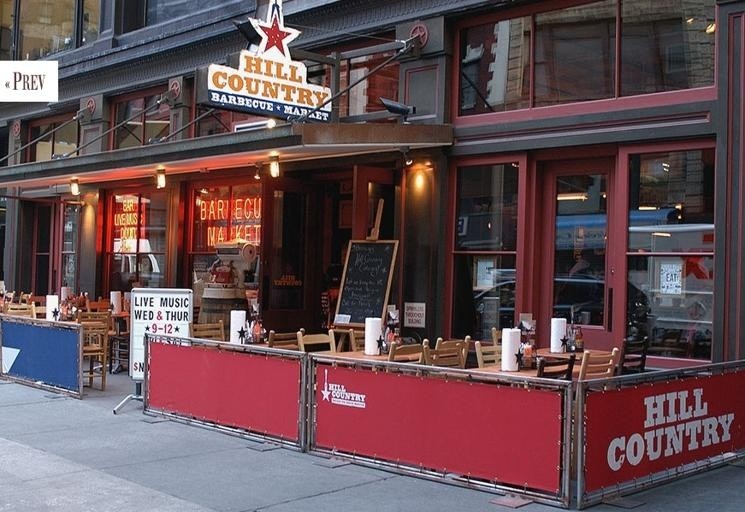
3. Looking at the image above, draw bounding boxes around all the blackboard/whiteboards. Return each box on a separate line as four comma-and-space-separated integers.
333, 239, 399, 327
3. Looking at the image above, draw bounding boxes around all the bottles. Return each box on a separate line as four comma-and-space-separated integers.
576, 326, 584, 352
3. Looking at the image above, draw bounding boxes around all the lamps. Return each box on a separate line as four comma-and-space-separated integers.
156, 168, 166, 190
68, 178, 81, 198
398, 145, 413, 167
378, 95, 410, 125
252, 155, 280, 180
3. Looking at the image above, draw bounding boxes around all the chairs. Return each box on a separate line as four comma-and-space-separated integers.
187, 320, 647, 377
0, 289, 198, 391
